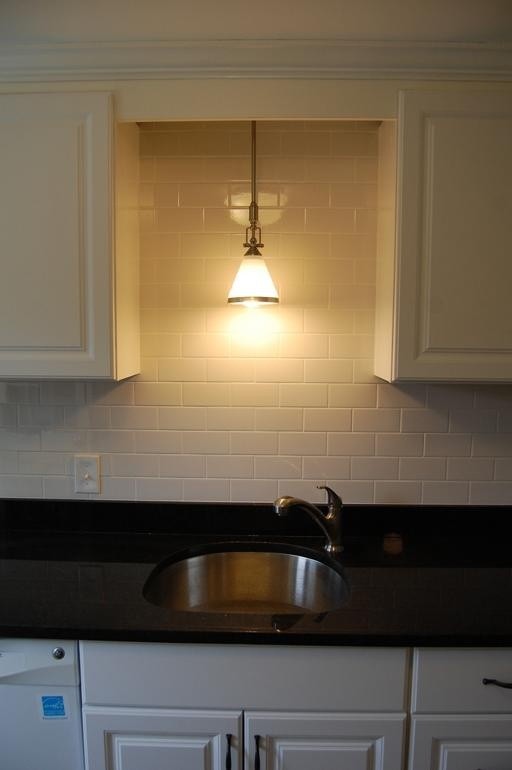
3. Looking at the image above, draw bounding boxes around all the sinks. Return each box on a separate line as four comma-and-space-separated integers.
142, 539, 350, 618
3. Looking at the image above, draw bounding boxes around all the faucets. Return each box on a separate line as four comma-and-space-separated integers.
273, 484, 344, 555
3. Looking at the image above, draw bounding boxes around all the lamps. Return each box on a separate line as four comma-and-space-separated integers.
226, 121, 281, 308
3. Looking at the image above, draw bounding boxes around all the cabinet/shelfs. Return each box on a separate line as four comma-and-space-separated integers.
406, 647, 512, 769
373, 87, 511, 386
77, 639, 410, 770
1, 90, 142, 383
